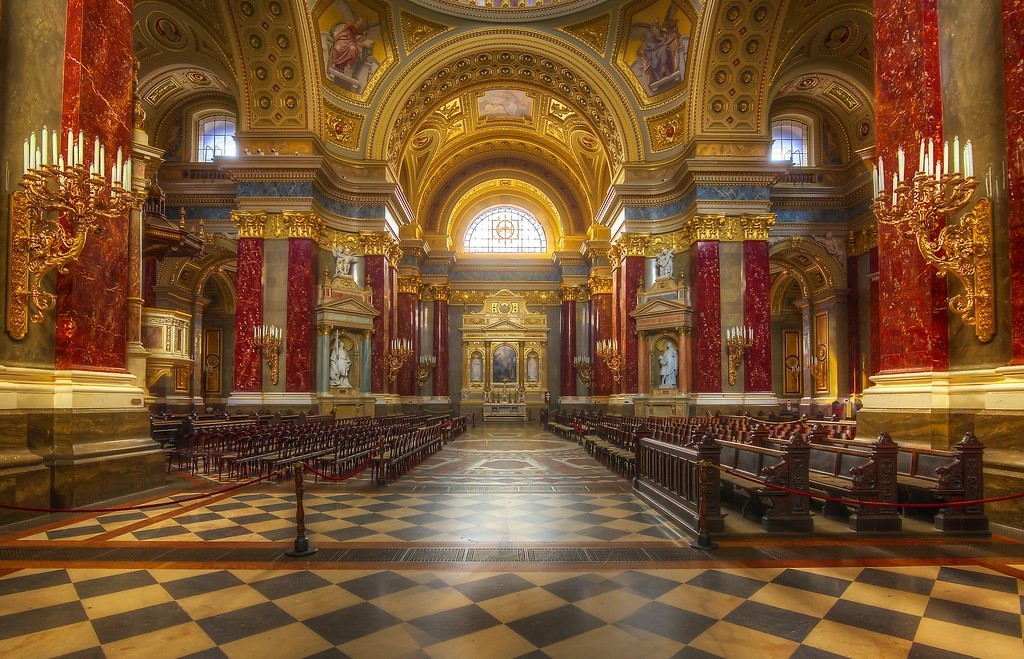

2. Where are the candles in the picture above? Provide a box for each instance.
[935,160,941,193]
[42,125,48,170]
[254,325,282,339]
[597,339,617,350]
[392,337,412,350]
[727,326,753,340]
[52,129,57,164]
[68,128,83,174]
[892,149,905,205]
[420,354,436,363]
[964,139,974,181]
[574,355,590,363]
[90,136,104,197]
[873,155,884,202]
[111,146,132,197]
[954,135,959,174]
[913,137,933,202]
[24,125,41,184]
[944,140,949,174]
[58,154,65,190]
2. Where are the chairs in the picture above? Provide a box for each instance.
[548,415,854,481]
[168,415,467,485]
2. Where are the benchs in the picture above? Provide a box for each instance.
[682,422,813,532]
[808,424,989,533]
[748,424,901,532]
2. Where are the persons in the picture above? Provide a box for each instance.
[336,246,352,275]
[330,342,353,388]
[656,249,675,276]
[659,342,679,385]
